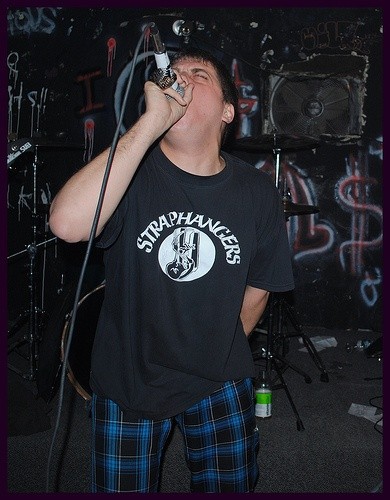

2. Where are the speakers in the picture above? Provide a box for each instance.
[264,62,365,142]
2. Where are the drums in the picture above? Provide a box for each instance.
[61,283,106,400]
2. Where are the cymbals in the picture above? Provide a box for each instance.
[225,133,321,154]
[282,199,320,216]
[32,137,85,147]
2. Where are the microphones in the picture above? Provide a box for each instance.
[150,23,185,101]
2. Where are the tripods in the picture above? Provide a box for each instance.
[247,138,329,432]
[3,105,58,382]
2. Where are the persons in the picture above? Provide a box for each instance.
[49,53,297,495]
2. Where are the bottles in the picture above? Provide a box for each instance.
[348,338,374,351]
[253,369,273,420]
